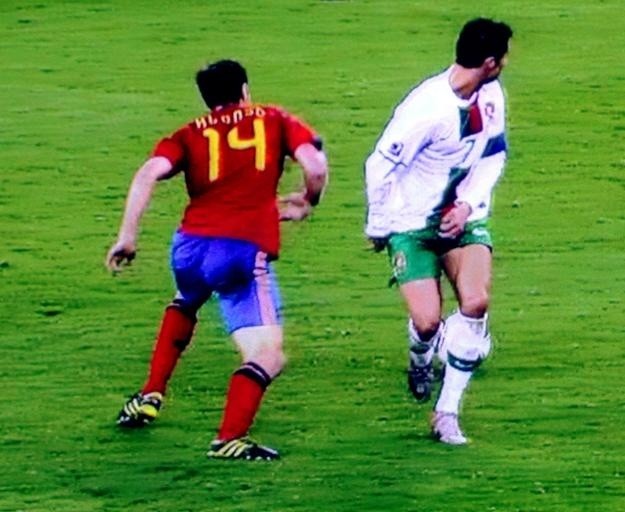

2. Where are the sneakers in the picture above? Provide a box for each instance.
[408,361,434,400]
[430,410,467,445]
[206,437,279,461]
[117,391,164,426]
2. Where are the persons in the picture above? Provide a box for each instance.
[365,19,513,445]
[106,59,327,460]
